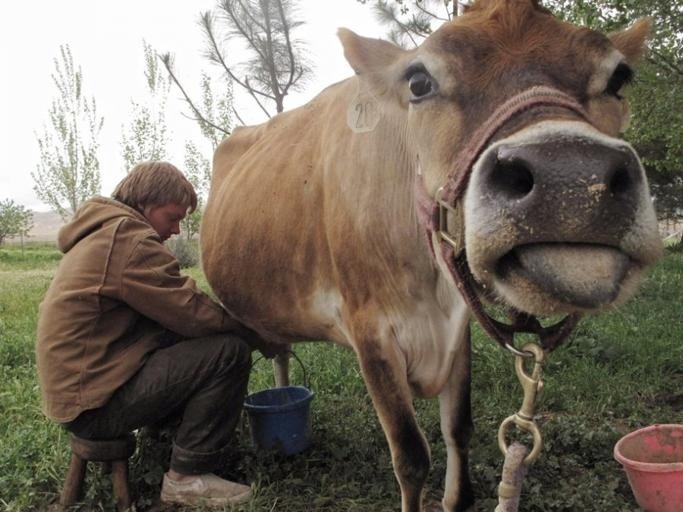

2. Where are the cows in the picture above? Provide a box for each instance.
[197,1,667,512]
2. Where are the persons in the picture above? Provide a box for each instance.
[36,158,276,510]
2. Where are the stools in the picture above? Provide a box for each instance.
[59,431,136,512]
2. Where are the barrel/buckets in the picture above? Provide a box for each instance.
[245,349,316,467]
[613,422,683,511]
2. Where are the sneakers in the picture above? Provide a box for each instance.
[162,473,253,506]
